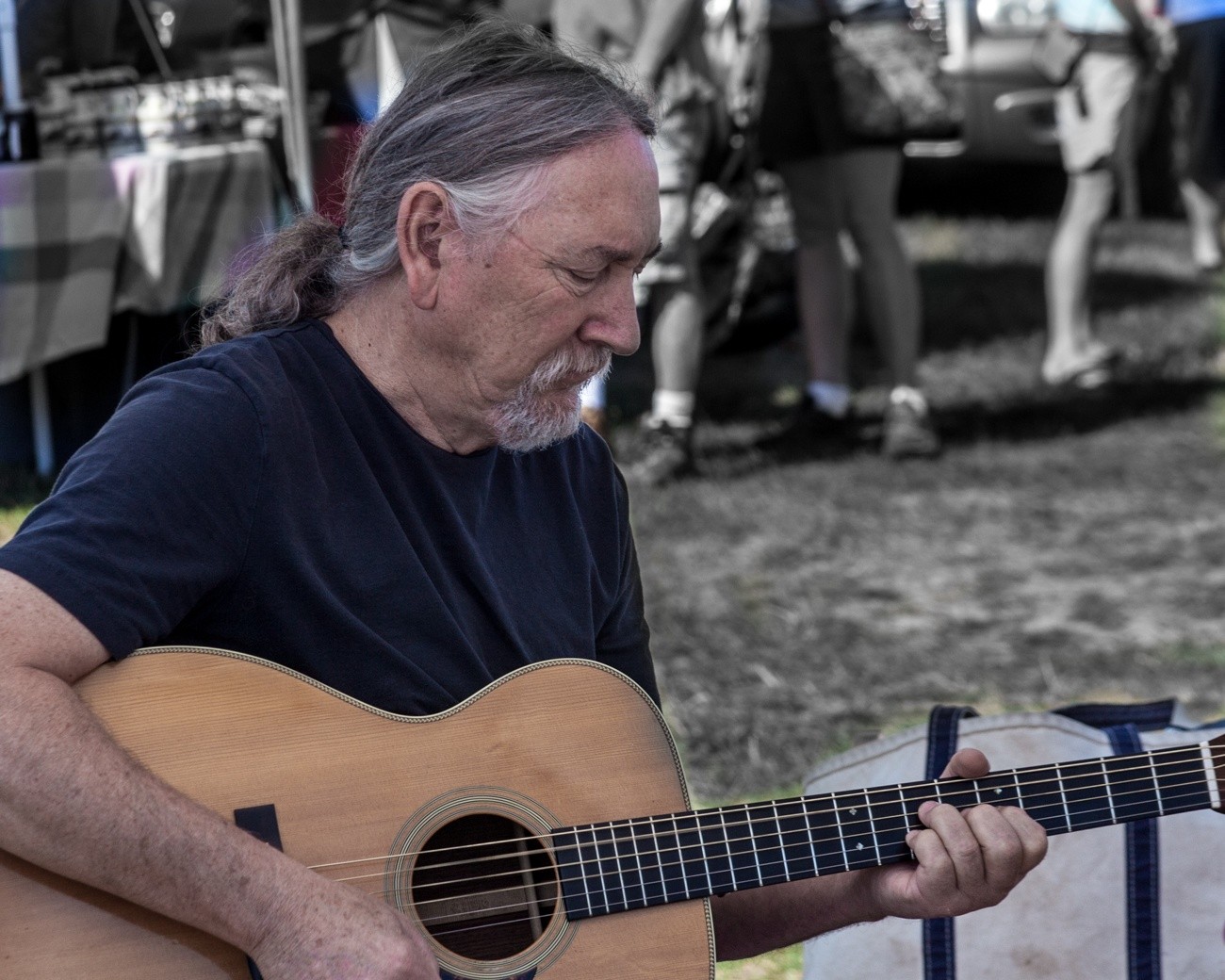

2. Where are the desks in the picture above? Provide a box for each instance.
[0,140,301,480]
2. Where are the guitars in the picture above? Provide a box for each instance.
[0,642,1224,978]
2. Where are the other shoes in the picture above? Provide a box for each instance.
[752,392,860,460]
[617,418,692,485]
[881,385,942,457]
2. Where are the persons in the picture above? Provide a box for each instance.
[0,21,1049,980]
[532,0,1225,487]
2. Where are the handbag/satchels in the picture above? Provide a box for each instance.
[1030,18,1083,87]
